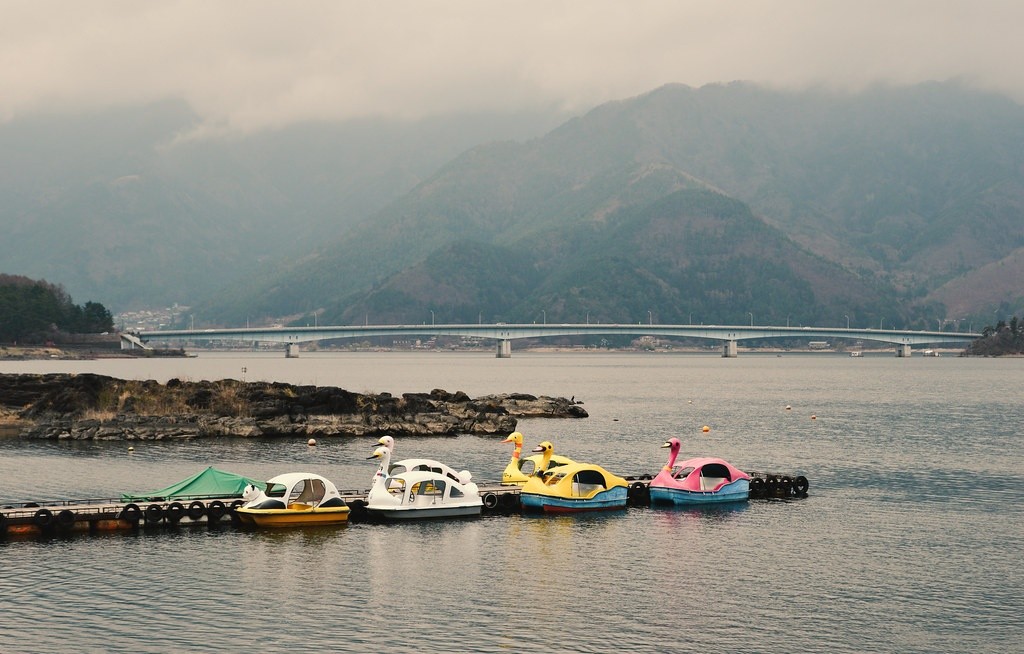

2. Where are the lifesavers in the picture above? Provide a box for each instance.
[631,482,647,502]
[482,493,499,509]
[779,476,793,495]
[751,477,766,494]
[56,510,76,531]
[765,474,779,494]
[188,501,206,520]
[146,504,163,522]
[639,473,652,480]
[24,502,39,508]
[351,499,367,516]
[229,500,245,517]
[35,508,54,528]
[208,501,226,520]
[793,475,810,495]
[500,493,517,509]
[122,503,142,525]
[167,502,186,522]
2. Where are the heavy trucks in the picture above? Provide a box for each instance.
[808,342,831,350]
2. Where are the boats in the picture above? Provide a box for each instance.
[649,438,752,505]
[850,351,864,356]
[350,436,485,524]
[233,472,349,524]
[499,432,579,490]
[521,441,629,513]
